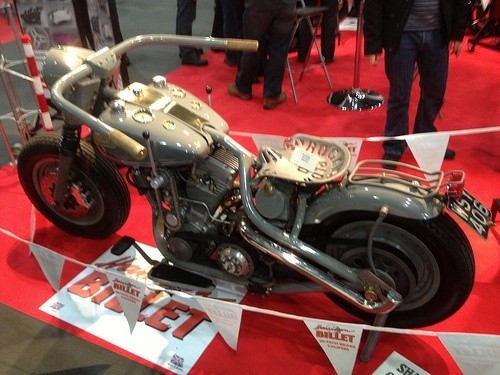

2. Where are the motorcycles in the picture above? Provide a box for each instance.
[0,33,492,361]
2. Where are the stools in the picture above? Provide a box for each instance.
[286,0,333,103]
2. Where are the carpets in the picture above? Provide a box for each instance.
[0,14,500,375]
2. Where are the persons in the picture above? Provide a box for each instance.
[362,0,471,170]
[296,0,339,62]
[176,0,268,83]
[228,0,296,109]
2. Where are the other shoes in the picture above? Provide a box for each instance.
[179,47,203,57]
[445,149,456,158]
[124,56,130,65]
[263,92,286,109]
[382,154,401,169]
[230,85,253,100]
[182,57,208,65]
